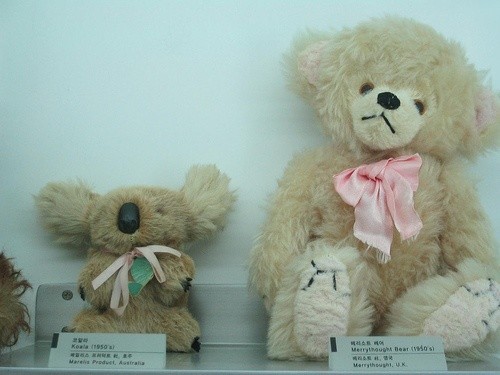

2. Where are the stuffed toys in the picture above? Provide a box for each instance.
[35,162,238,352]
[247,11,500,362]
